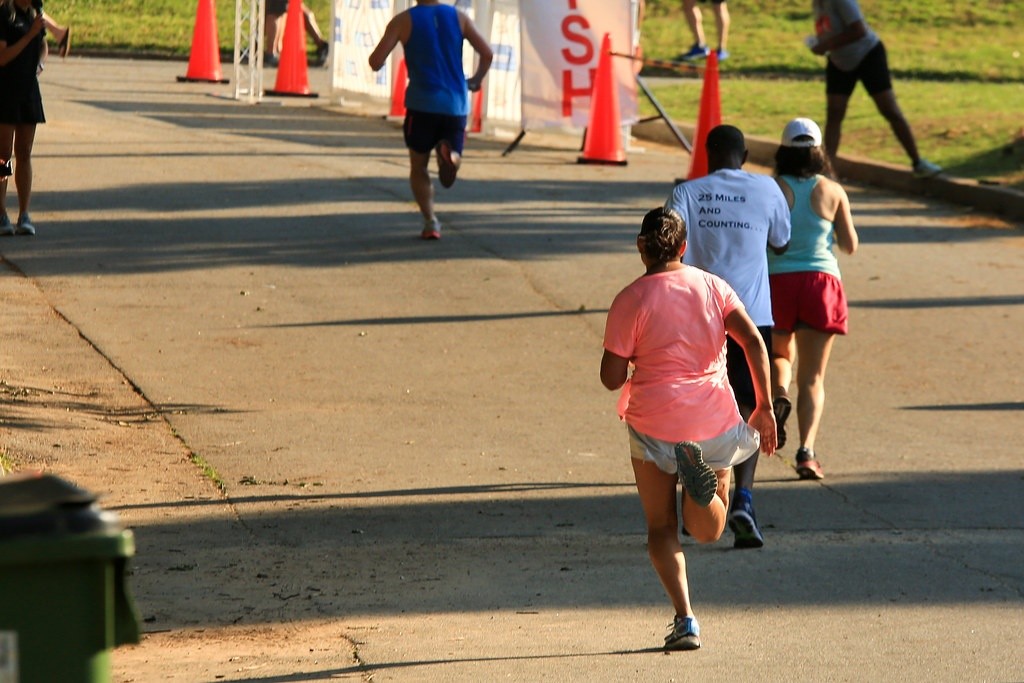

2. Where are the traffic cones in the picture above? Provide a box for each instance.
[391,59,409,116]
[675,50,722,184]
[576,33,628,167]
[470,84,483,133]
[176,0,229,85]
[264,0,319,98]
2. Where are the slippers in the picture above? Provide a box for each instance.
[59,26,69,59]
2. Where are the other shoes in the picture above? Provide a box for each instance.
[0,215,15,236]
[264,52,279,68]
[17,219,35,235]
[315,42,328,67]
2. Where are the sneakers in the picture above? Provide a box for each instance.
[677,43,710,62]
[436,139,457,189]
[663,615,701,649]
[728,488,764,548]
[422,220,441,239]
[715,50,730,61]
[795,447,825,479]
[675,440,718,508]
[913,159,941,179]
[773,396,792,450]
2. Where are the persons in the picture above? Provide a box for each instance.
[768,117,859,480]
[0,0,72,236]
[811,0,944,179]
[664,125,793,547]
[257,0,329,68]
[600,207,778,652]
[681,0,730,61]
[368,0,494,241]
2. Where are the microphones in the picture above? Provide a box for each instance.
[31,0,46,36]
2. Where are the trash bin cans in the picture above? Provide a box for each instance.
[0,475,140,683]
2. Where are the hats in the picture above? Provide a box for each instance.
[781,118,822,147]
